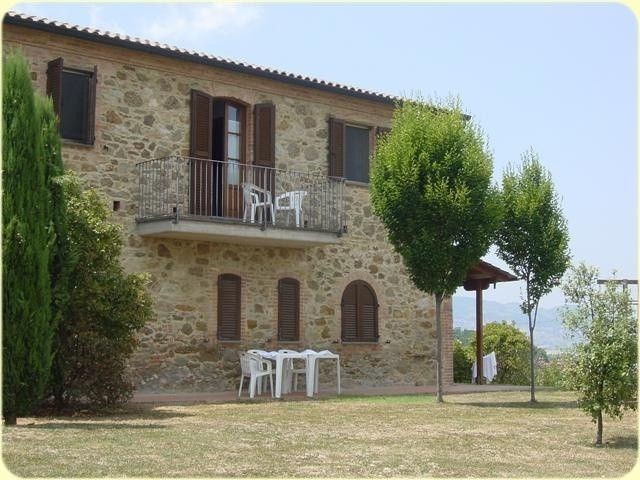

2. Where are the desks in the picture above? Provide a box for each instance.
[275,354,340,399]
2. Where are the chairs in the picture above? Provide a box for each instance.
[272,191,307,227]
[278,350,306,393]
[242,183,275,225]
[247,350,276,393]
[236,351,274,398]
